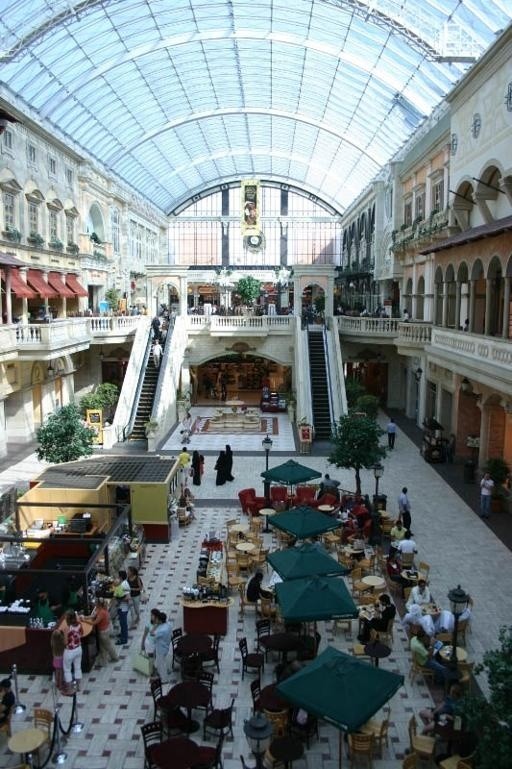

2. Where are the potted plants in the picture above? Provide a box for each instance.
[482,458,508,513]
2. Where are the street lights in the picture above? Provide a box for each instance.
[441,583,470,697]
[367,461,384,546]
[263,288,269,314]
[261,432,274,504]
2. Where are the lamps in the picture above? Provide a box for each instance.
[100,344,104,360]
[415,368,423,379]
[462,377,470,394]
[47,360,54,375]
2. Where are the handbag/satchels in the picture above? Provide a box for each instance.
[141,593,149,601]
[133,652,153,675]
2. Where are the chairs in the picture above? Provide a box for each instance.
[0,704,16,737]
[34,709,53,750]
[68,310,122,328]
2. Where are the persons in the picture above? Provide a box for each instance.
[359,303,386,318]
[150,306,177,370]
[50,304,147,319]
[187,301,325,325]
[26,444,234,695]
[402,309,409,323]
[338,304,344,314]
[317,416,493,667]
[245,570,272,604]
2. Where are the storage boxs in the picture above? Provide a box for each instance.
[70,513,90,533]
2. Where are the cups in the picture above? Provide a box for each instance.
[54,524,64,534]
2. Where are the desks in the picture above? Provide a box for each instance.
[465,442,479,456]
[8,728,43,769]
[421,439,441,462]
[260,400,287,412]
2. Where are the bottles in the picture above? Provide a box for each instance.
[29,617,45,629]
